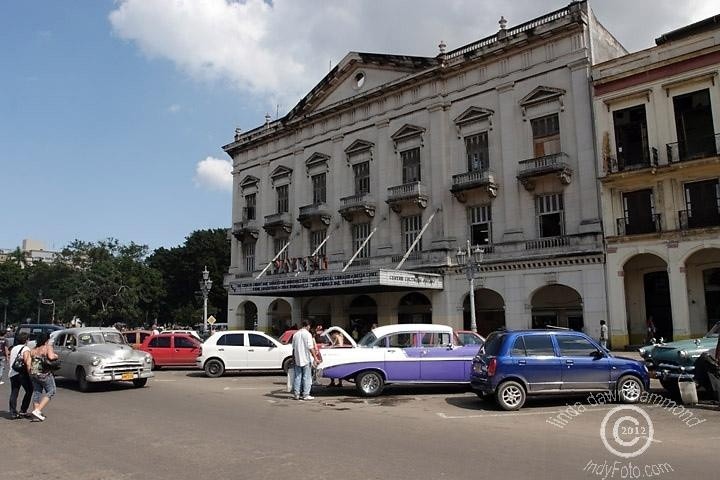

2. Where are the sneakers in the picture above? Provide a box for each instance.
[19,413,30,418]
[31,409,45,422]
[303,395,314,400]
[11,413,18,418]
[295,394,300,400]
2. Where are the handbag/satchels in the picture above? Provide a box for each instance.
[41,356,63,371]
[12,358,27,373]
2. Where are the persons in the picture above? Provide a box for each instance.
[112,321,228,343]
[285,318,380,400]
[598,319,608,350]
[0,323,58,422]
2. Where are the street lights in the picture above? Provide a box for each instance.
[456,240,488,336]
[197,265,214,333]
